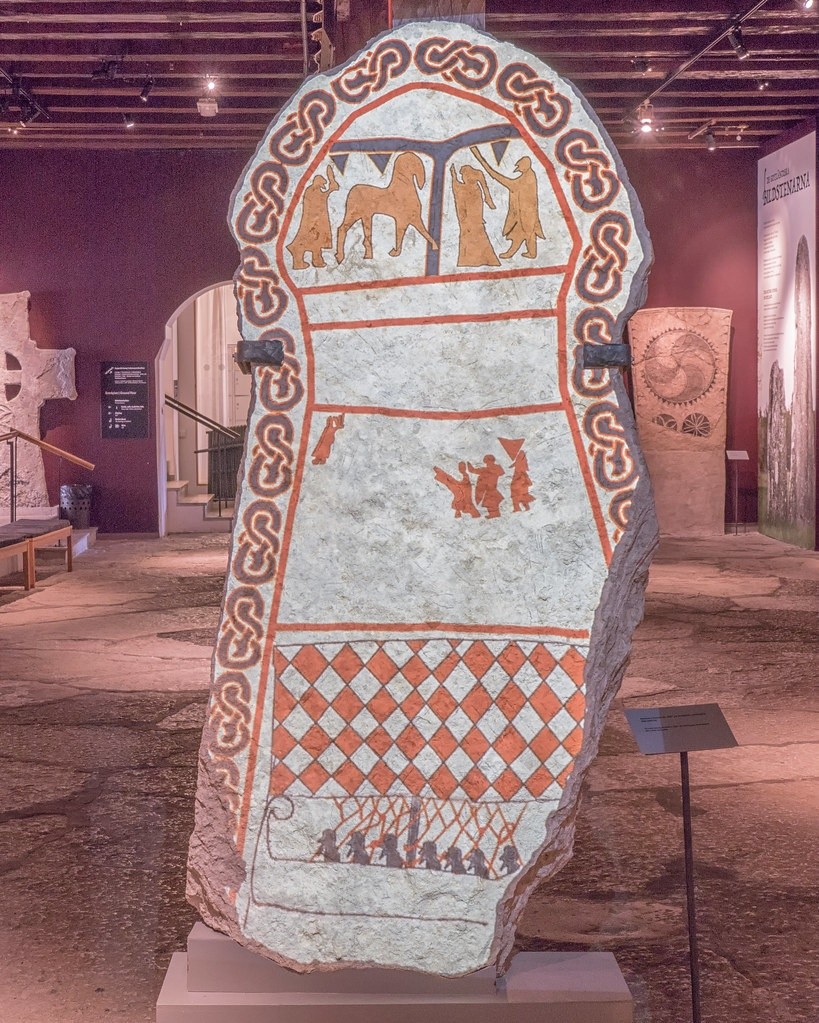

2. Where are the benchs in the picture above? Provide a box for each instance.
[0,518,74,590]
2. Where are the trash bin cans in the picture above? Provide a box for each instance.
[59,484,93,530]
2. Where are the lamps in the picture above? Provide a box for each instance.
[197,98,219,117]
[622,119,635,135]
[20,110,40,128]
[728,34,749,60]
[795,0,813,8]
[705,132,716,151]
[138,79,153,102]
[637,101,654,132]
[121,112,134,129]
[634,61,651,75]
